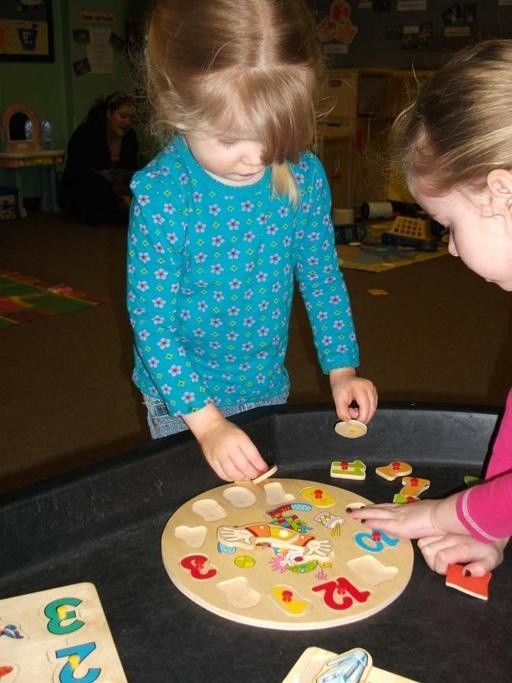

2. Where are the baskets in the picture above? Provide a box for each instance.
[386,215,436,242]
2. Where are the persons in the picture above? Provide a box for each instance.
[58,89,138,228]
[345,37,512,581]
[124,1,379,480]
